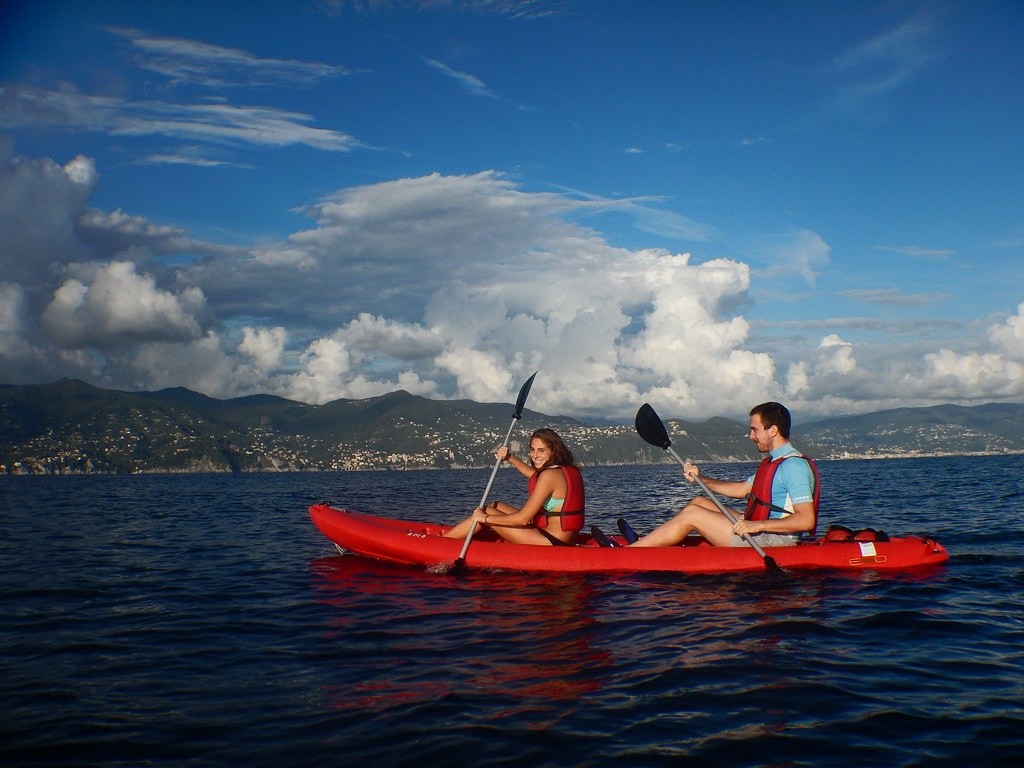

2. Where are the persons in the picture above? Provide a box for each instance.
[443,428,584,546]
[590,402,820,548]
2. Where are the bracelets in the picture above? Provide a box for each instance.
[484,514,491,523]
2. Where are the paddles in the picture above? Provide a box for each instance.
[634,402,785,575]
[447,370,540,570]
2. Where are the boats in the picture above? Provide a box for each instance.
[307,501,949,583]
